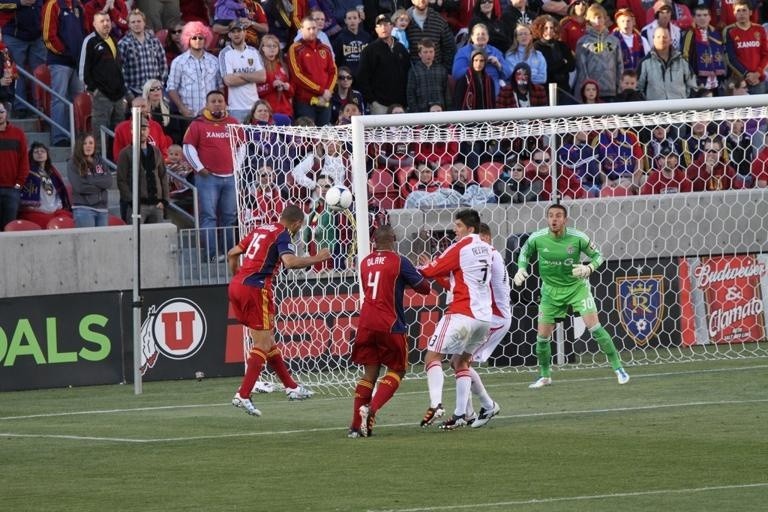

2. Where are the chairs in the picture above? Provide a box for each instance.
[0,43,767,232]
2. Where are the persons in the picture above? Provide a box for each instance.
[415,210,493,430]
[514,204,630,388]
[0,1,767,273]
[229,206,331,417]
[346,226,431,438]
[465,223,511,427]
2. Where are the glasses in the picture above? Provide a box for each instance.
[316,185,331,189]
[192,34,205,40]
[574,1,587,6]
[706,147,721,153]
[535,158,550,164]
[260,172,273,177]
[149,86,162,91]
[337,75,354,81]
[512,168,523,172]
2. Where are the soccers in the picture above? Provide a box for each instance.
[324,183,354,213]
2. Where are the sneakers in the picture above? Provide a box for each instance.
[420,403,446,429]
[231,391,263,418]
[438,413,468,432]
[347,428,360,438]
[466,411,478,424]
[285,385,314,401]
[528,376,552,389]
[613,366,631,384]
[15,110,27,118]
[359,404,375,438]
[54,139,71,147]
[471,401,500,428]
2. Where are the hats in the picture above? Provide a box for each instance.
[130,117,150,129]
[566,0,582,13]
[658,146,678,158]
[375,14,391,25]
[614,8,636,19]
[228,20,245,32]
[652,0,673,14]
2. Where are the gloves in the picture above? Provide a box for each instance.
[514,267,530,286]
[571,264,594,279]
